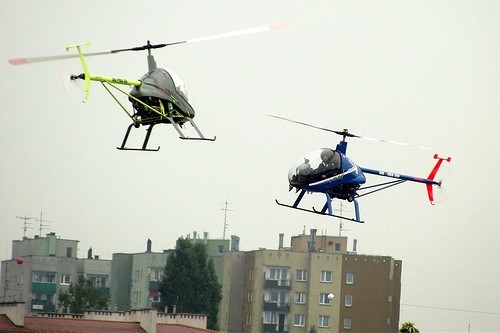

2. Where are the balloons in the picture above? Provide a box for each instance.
[15,256,25,265]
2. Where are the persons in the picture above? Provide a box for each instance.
[311,151,337,172]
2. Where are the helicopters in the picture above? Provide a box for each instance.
[6,22,288,152]
[262,113,452,223]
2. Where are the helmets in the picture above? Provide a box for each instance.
[320,150,333,159]
